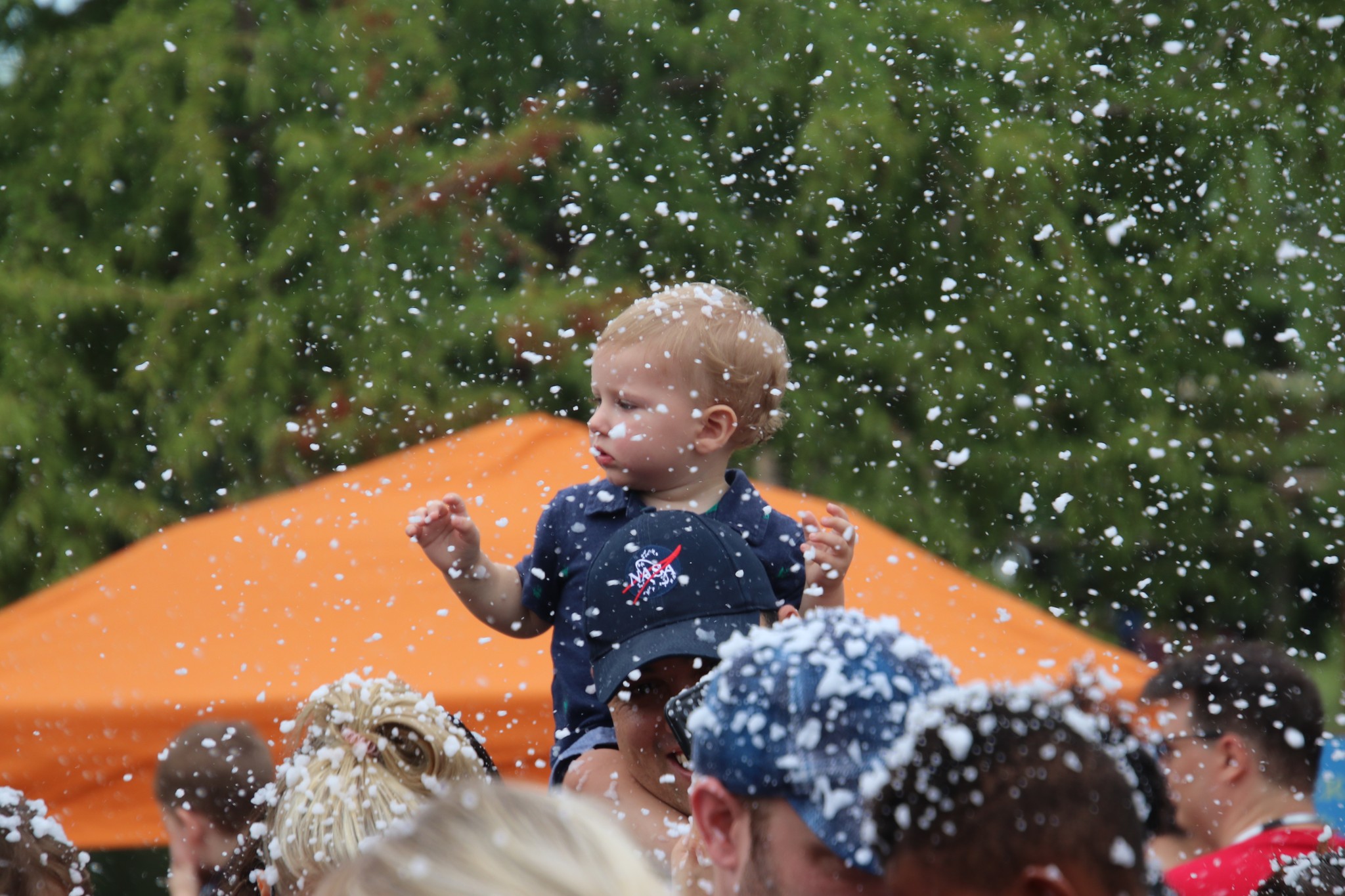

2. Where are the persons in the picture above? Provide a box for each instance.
[0,608,1345,896]
[409,280,858,882]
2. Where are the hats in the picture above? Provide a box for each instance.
[581,506,777,705]
[689,610,957,877]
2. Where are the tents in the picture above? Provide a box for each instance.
[0,413,1167,851]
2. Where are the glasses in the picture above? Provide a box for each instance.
[1147,730,1222,760]
